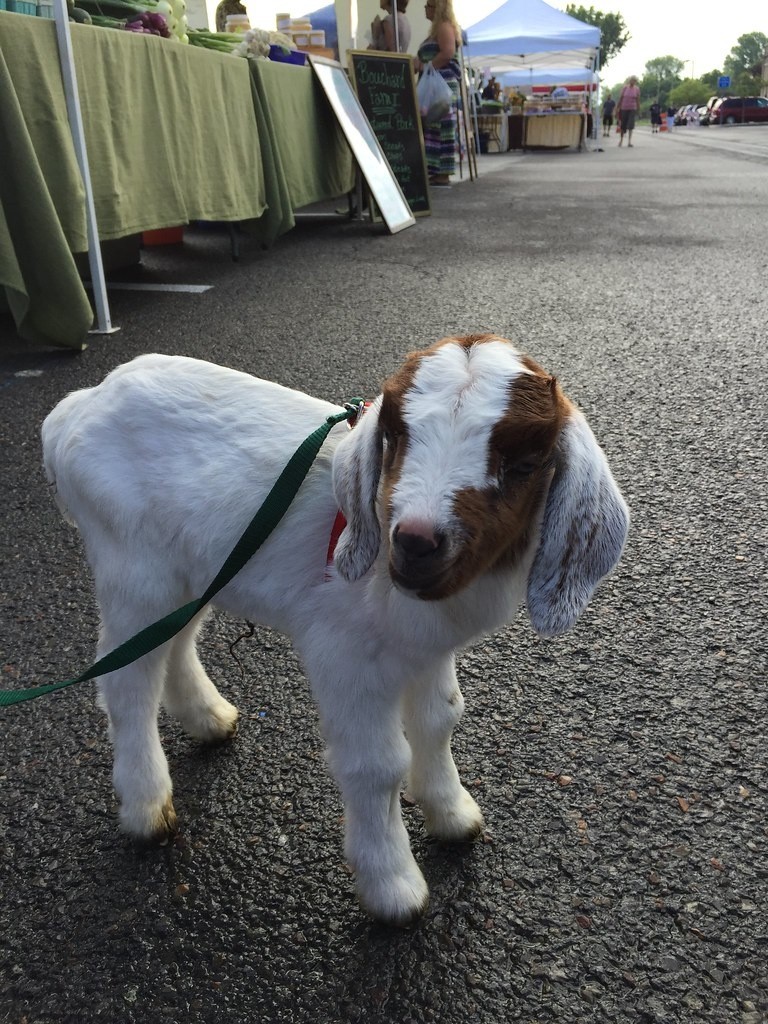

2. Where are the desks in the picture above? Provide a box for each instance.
[467,96,595,151]
[0,8,364,223]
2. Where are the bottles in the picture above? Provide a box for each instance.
[226,9,329,49]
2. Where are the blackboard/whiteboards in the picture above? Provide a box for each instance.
[307,53,416,234]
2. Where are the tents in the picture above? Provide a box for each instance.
[459,0,602,152]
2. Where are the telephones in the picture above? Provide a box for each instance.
[345,48,432,222]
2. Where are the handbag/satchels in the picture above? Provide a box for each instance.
[615,104,627,134]
[414,60,454,130]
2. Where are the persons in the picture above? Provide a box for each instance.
[665,102,681,133]
[617,77,641,147]
[600,92,615,138]
[649,99,662,134]
[409,0,462,189]
[372,1,413,53]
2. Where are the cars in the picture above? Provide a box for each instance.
[672,93,768,127]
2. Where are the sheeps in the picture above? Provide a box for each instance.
[42,335,630,925]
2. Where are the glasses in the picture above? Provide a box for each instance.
[424,2,436,9]
